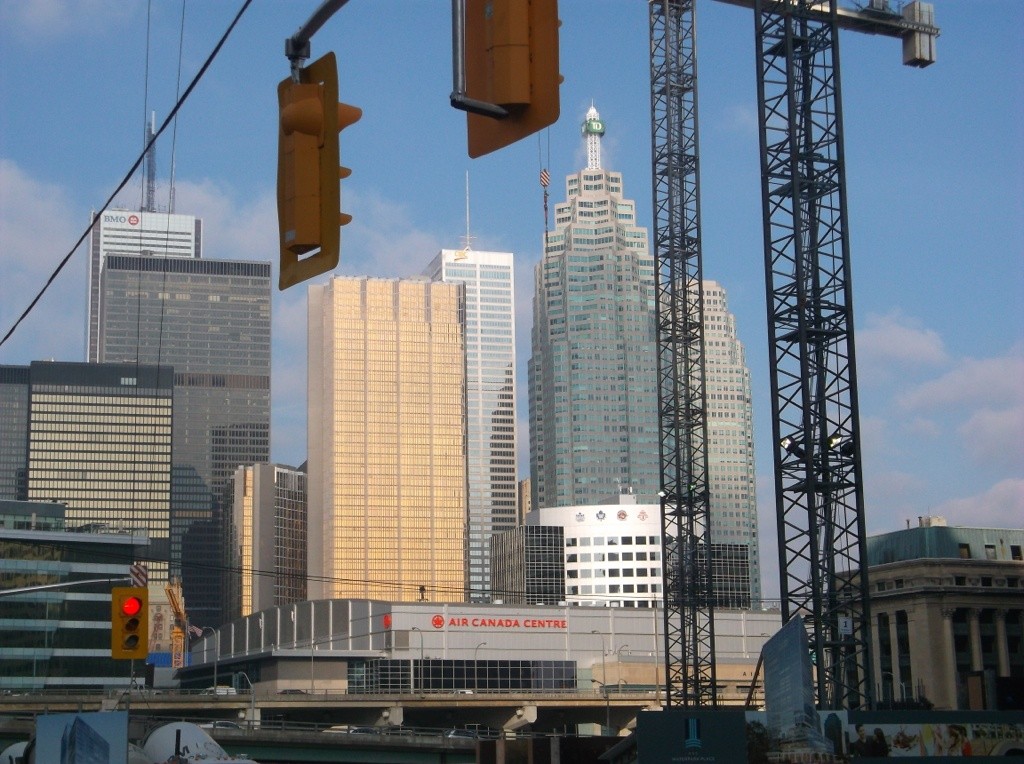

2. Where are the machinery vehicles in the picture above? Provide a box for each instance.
[0,721,257,764]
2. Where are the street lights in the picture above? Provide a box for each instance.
[883,672,906,704]
[202,626,217,695]
[412,627,425,694]
[591,679,610,737]
[592,630,606,686]
[474,643,488,694]
[617,645,629,694]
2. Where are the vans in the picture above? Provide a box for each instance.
[200,684,236,694]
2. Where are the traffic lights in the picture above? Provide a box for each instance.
[112,587,150,659]
[465,0,565,159]
[275,49,364,291]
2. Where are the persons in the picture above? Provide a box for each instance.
[853,723,874,757]
[872,727,889,757]
[974,726,988,753]
[749,745,769,764]
[934,725,944,752]
[945,725,972,756]
[892,730,911,750]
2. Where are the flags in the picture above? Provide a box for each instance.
[188,622,203,637]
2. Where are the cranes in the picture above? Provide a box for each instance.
[649,0,720,704]
[729,1,941,708]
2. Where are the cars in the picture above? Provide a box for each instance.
[277,689,308,694]
[116,684,163,695]
[449,689,473,695]
[197,721,240,730]
[320,726,386,736]
[437,728,485,739]
